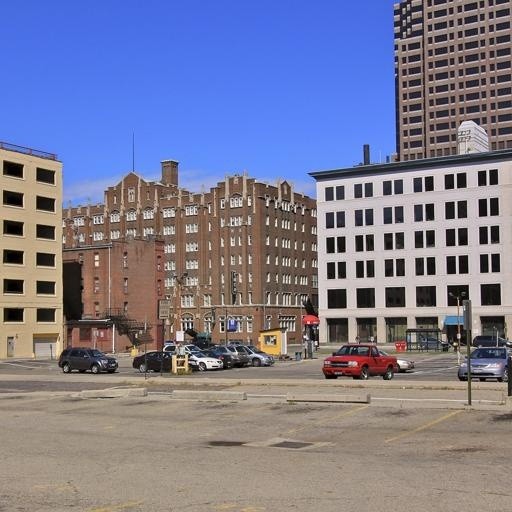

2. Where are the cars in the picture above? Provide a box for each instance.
[379,349,414,372]
[456,346,510,382]
[421,336,451,349]
[133,336,274,375]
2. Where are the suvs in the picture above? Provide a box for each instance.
[57,346,119,375]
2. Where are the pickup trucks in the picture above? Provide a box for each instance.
[321,343,398,380]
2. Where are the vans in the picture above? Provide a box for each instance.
[470,334,512,347]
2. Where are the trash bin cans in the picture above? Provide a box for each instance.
[295,352,302,361]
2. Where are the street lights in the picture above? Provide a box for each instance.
[492,325,500,347]
[448,292,462,368]
